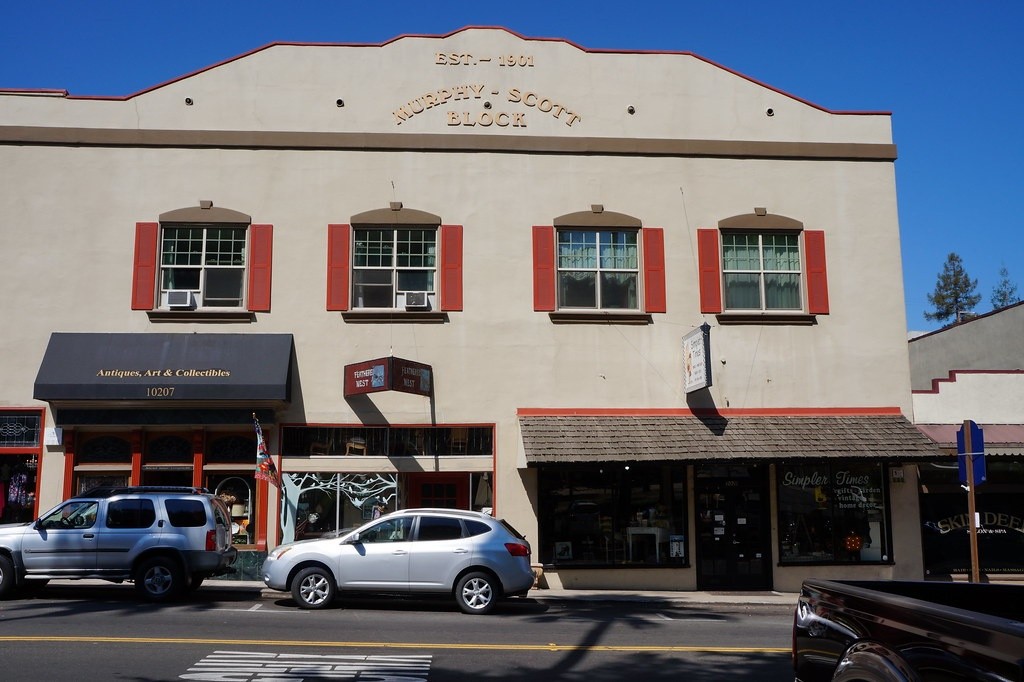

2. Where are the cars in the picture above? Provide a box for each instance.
[262,507,538,611]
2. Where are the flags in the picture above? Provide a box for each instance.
[254,418,278,488]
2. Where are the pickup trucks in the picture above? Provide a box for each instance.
[795,581,1024,682]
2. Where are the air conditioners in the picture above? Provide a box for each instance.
[405,292,427,307]
[166,290,194,307]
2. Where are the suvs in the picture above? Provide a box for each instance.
[0,484,238,607]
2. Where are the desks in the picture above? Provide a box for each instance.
[227,544,266,580]
[627,527,671,562]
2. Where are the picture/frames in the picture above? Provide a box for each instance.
[554,541,573,559]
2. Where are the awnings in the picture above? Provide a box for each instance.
[515,412,1024,469]
[34,332,292,399]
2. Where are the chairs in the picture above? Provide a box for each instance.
[346,433,372,455]
[598,515,626,563]
[449,429,468,455]
[310,429,335,455]
